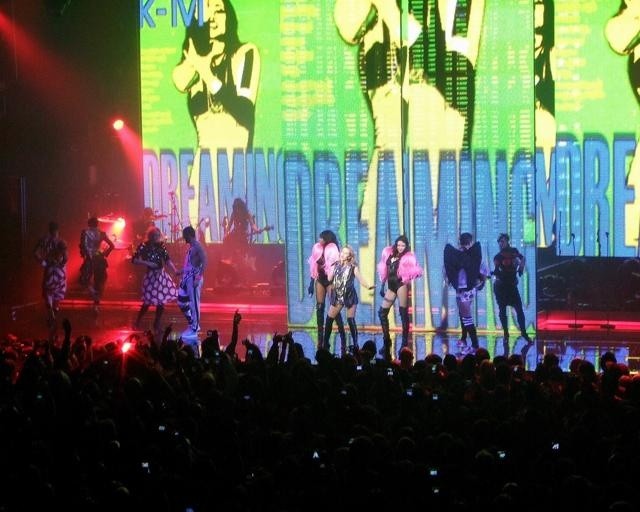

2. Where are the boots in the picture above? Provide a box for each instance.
[316,302,346,352]
[347,316,359,352]
[399,306,408,354]
[378,306,392,354]
[499,316,509,344]
[135,303,163,329]
[516,314,532,343]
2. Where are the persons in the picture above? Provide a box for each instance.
[33,218,115,325]
[225,198,259,269]
[143,208,155,241]
[444,233,485,355]
[309,230,376,351]
[490,233,533,344]
[173,226,206,336]
[376,236,422,356]
[131,227,177,332]
[1,309,639,512]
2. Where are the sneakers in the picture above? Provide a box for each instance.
[456,340,477,355]
[182,330,197,338]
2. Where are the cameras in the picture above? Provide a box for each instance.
[139,364,560,494]
